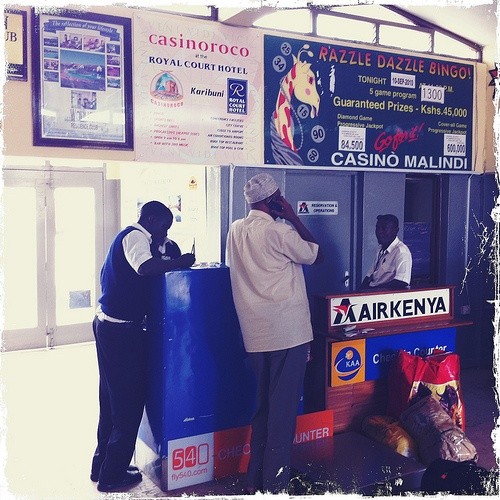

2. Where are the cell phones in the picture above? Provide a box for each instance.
[268,197,282,213]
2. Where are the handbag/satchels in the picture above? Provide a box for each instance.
[366,346,481,465]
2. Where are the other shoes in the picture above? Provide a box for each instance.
[90,464,142,492]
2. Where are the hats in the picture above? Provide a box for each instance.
[243,173,279,204]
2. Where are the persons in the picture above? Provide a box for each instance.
[44,34,120,88]
[137,202,143,219]
[359,214,412,289]
[78,96,96,109]
[167,199,181,222]
[90,200,196,491]
[157,230,182,261]
[225,173,323,496]
